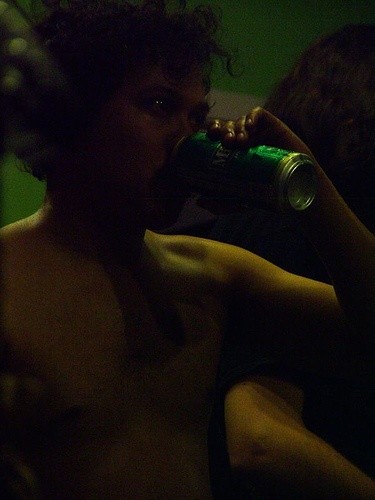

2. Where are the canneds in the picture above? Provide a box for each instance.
[171,128,319,212]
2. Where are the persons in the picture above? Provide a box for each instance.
[0,0,375,499]
[209,20,375,498]
